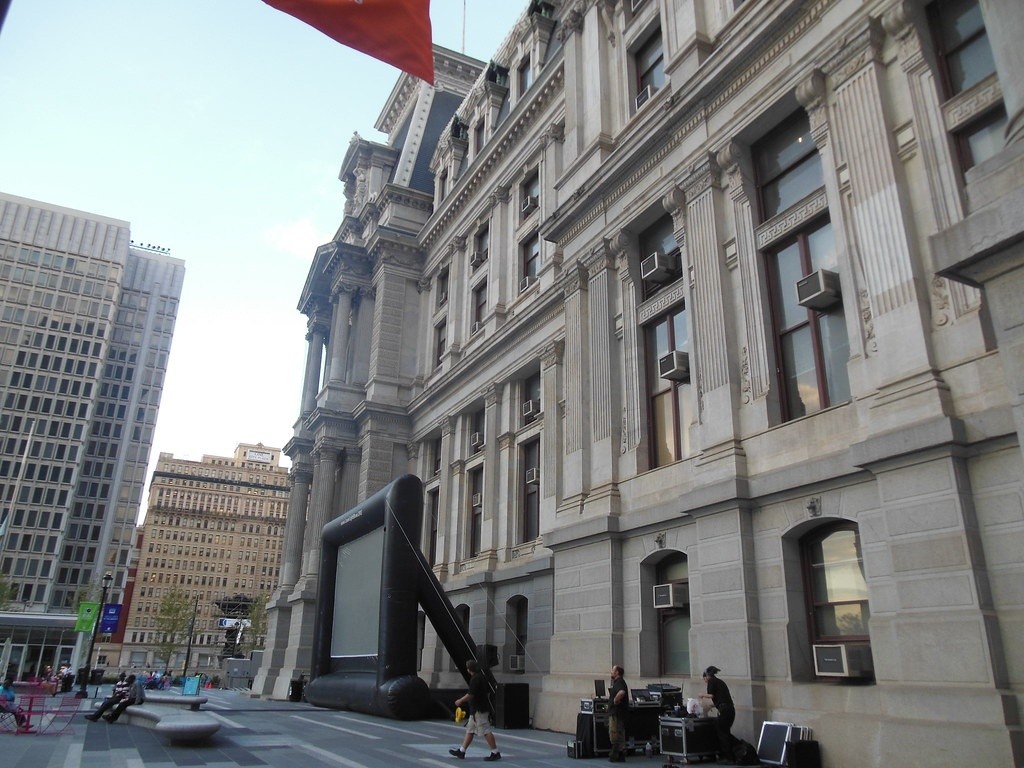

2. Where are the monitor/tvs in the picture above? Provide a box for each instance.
[595,680,605,698]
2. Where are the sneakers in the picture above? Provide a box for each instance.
[449,748,465,759]
[484,752,501,761]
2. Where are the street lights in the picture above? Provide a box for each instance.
[182,595,199,676]
[75,571,113,698]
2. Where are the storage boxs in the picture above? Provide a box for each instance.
[631,688,661,707]
[658,715,721,764]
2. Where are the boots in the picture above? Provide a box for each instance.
[616,751,625,762]
[609,743,619,762]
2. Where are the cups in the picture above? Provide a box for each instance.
[674,706,680,712]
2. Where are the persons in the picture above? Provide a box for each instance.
[85,673,130,722]
[102,675,145,724]
[608,665,628,762]
[698,666,736,765]
[0,677,33,729]
[146,670,173,690]
[62,664,72,676]
[449,660,501,761]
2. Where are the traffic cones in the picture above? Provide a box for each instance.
[204,682,212,688]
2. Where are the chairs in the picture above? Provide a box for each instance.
[0,694,81,737]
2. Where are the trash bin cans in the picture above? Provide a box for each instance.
[289,679,304,702]
[91,668,105,685]
[78,668,84,684]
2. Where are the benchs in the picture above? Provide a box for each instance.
[94,701,221,746]
[105,693,208,711]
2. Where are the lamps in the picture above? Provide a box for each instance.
[634,84,659,112]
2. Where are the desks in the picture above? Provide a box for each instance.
[17,695,46,733]
[576,706,687,758]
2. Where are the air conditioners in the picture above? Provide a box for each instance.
[794,268,842,309]
[470,251,486,267]
[520,276,537,293]
[658,350,690,381]
[522,196,538,213]
[471,432,484,447]
[439,292,447,303]
[472,493,482,506]
[526,468,540,485]
[640,251,676,283]
[523,400,540,417]
[813,644,874,677]
[471,321,485,336]
[652,584,689,608]
[508,655,525,670]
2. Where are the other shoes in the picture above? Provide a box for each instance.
[85,715,97,722]
[719,759,735,765]
[102,715,113,723]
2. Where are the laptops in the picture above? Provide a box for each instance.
[630,687,651,704]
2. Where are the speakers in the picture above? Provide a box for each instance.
[786,740,821,768]
[475,642,498,668]
[495,683,530,729]
